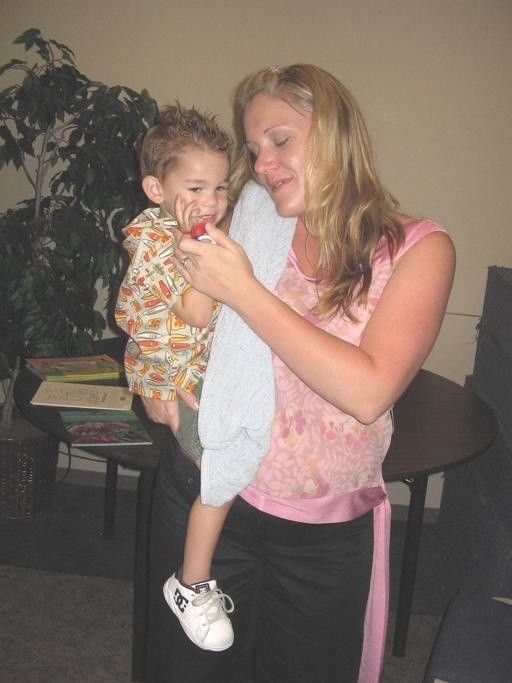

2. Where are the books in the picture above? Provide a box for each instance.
[59,408,153,447]
[26,354,122,382]
[30,379,134,410]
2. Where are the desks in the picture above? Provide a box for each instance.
[14,337,498,683]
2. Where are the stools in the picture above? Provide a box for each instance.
[425,589,512,683]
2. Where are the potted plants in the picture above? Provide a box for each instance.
[0,28,159,521]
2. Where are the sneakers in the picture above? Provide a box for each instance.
[162,572,235,652]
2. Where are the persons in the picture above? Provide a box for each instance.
[114,65,456,682]
